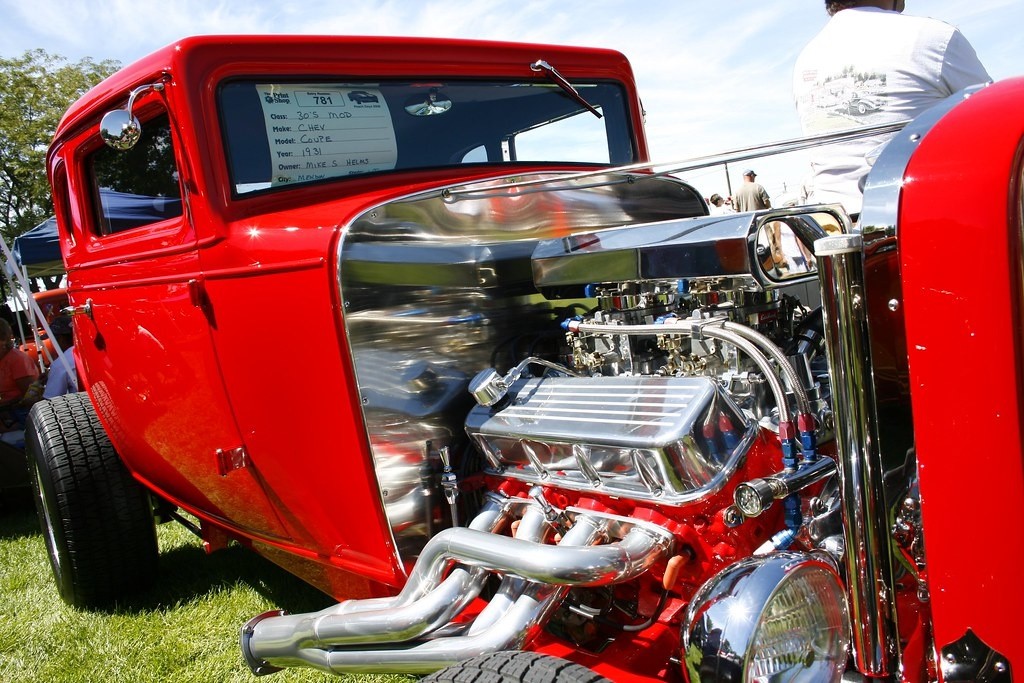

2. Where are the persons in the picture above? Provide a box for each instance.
[708,193,736,214]
[791,0,995,219]
[0,317,41,432]
[42,317,79,399]
[736,168,772,212]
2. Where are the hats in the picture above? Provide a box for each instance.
[744,169,757,176]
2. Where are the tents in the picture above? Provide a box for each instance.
[3,182,184,373]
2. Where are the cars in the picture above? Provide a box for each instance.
[0,27,1024,683]
